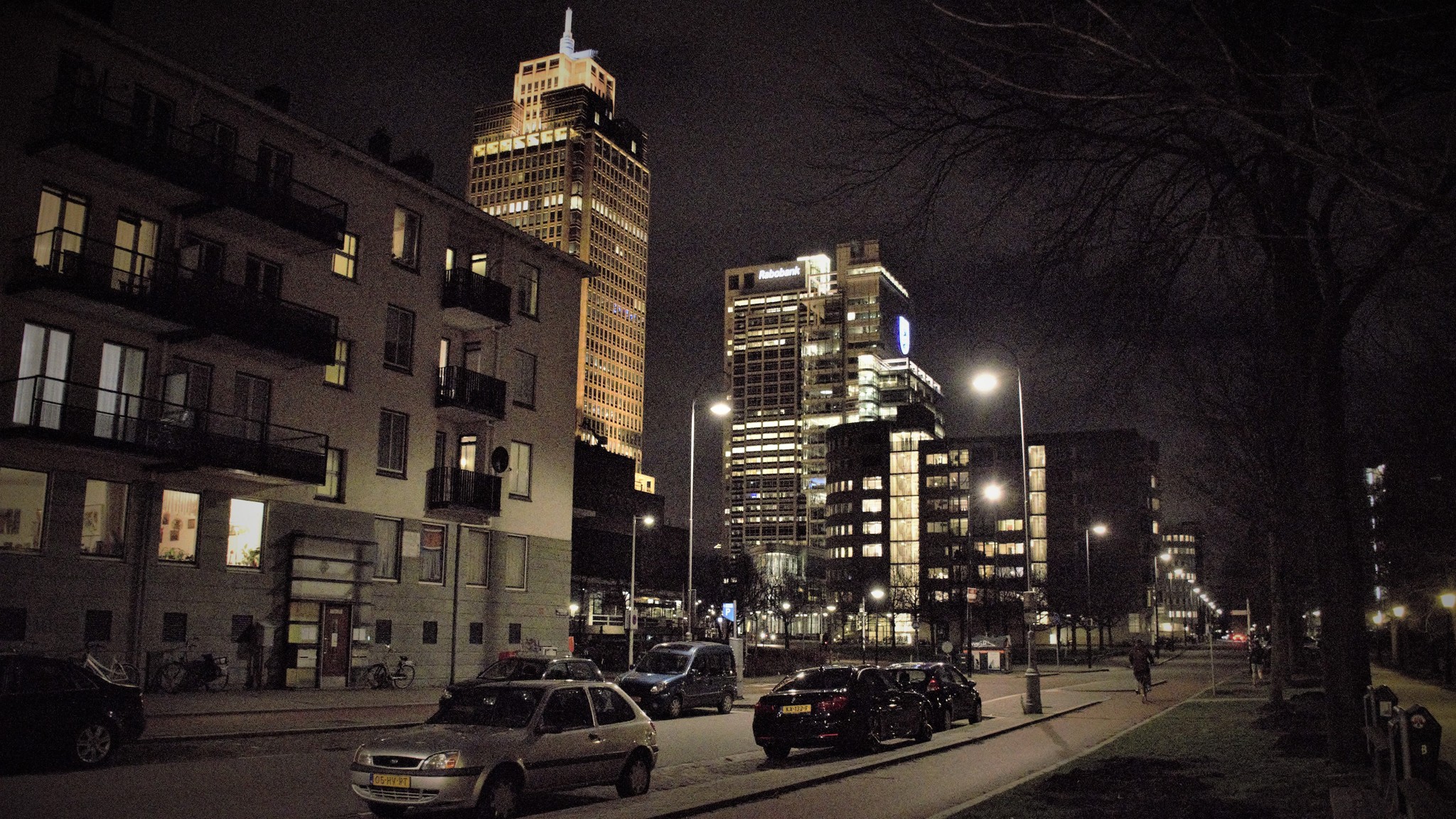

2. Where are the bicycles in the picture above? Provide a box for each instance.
[79,641,140,690]
[362,640,415,689]
[1130,662,1154,704]
[156,638,229,695]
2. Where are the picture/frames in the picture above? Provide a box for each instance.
[83,503,103,537]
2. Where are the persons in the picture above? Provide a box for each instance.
[1247,640,1266,687]
[1128,640,1153,695]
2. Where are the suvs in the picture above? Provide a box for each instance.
[620,641,740,720]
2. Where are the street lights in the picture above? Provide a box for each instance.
[1154,553,1223,660]
[688,393,732,639]
[629,514,654,671]
[1086,525,1107,668]
[862,588,884,664]
[966,338,1042,714]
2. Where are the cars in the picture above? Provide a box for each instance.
[0,653,146,770]
[438,654,608,719]
[885,662,983,733]
[350,678,659,819]
[751,664,934,760]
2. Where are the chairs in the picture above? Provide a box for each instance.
[899,672,910,682]
